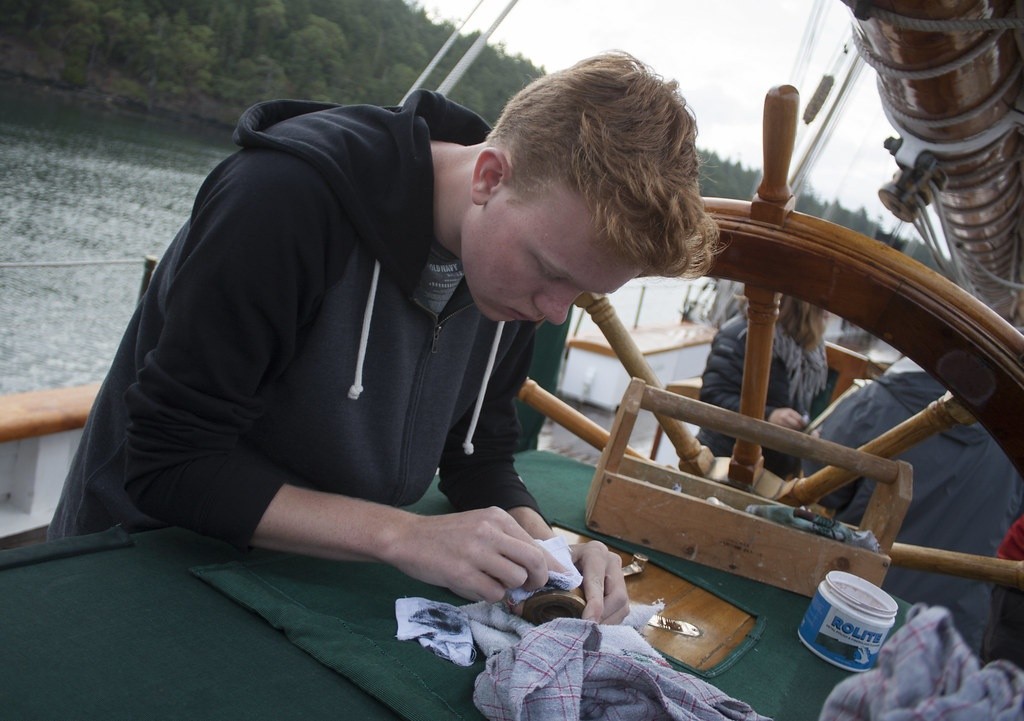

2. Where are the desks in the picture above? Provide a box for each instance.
[0,447,915,721]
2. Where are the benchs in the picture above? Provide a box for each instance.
[556,319,719,413]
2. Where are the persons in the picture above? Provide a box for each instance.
[799,357,1024,664]
[48,50,718,629]
[699,296,830,479]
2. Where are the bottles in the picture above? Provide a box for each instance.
[797,570,899,673]
